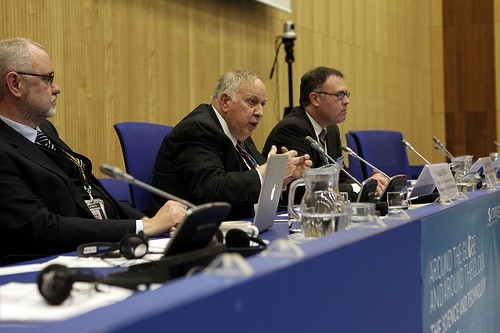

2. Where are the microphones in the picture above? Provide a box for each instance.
[340,143,393,178]
[402,139,429,164]
[432,136,453,157]
[98,163,225,242]
[433,145,453,159]
[305,135,361,186]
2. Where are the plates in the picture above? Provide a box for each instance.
[249,239,270,249]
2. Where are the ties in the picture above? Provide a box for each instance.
[235,141,257,170]
[317,129,327,153]
[35,131,84,171]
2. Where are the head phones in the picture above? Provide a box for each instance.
[78,231,149,259]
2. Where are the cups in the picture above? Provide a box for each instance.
[219,221,258,245]
[288,205,302,234]
[387,192,409,214]
[340,191,375,230]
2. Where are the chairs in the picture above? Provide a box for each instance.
[347,130,412,184]
[113,121,174,214]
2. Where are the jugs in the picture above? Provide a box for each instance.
[288,163,347,241]
[448,154,477,194]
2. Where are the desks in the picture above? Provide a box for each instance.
[0,182,500,333]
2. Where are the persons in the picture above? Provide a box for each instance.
[261,66,390,206]
[1,38,188,265]
[152,68,313,221]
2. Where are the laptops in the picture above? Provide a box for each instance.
[254,154,289,233]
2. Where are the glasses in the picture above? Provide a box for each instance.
[6,72,54,84]
[314,91,350,100]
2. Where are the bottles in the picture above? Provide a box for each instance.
[490,152,500,189]
